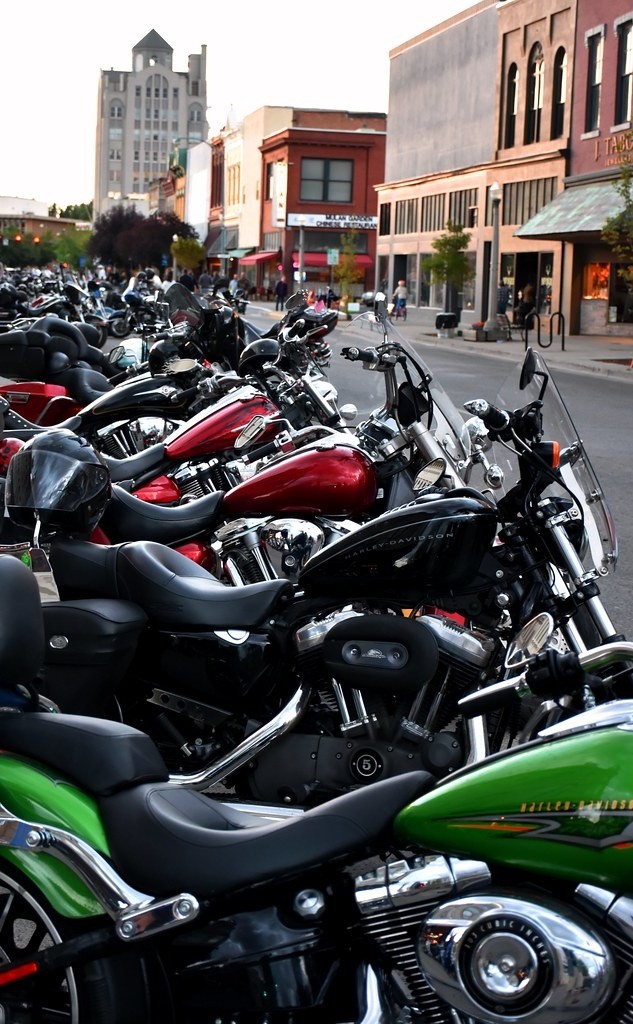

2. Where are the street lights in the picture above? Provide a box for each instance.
[483,180,503,333]
[297,214,306,290]
[172,233,180,281]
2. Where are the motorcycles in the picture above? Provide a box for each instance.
[0,249,359,507]
[1,339,632,814]
[0,552,632,1024]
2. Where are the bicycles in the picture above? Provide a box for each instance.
[393,294,407,322]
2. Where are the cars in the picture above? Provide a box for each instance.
[361,279,388,308]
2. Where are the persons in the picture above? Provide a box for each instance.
[522,282,536,322]
[3,259,338,310]
[495,281,510,318]
[393,278,408,313]
